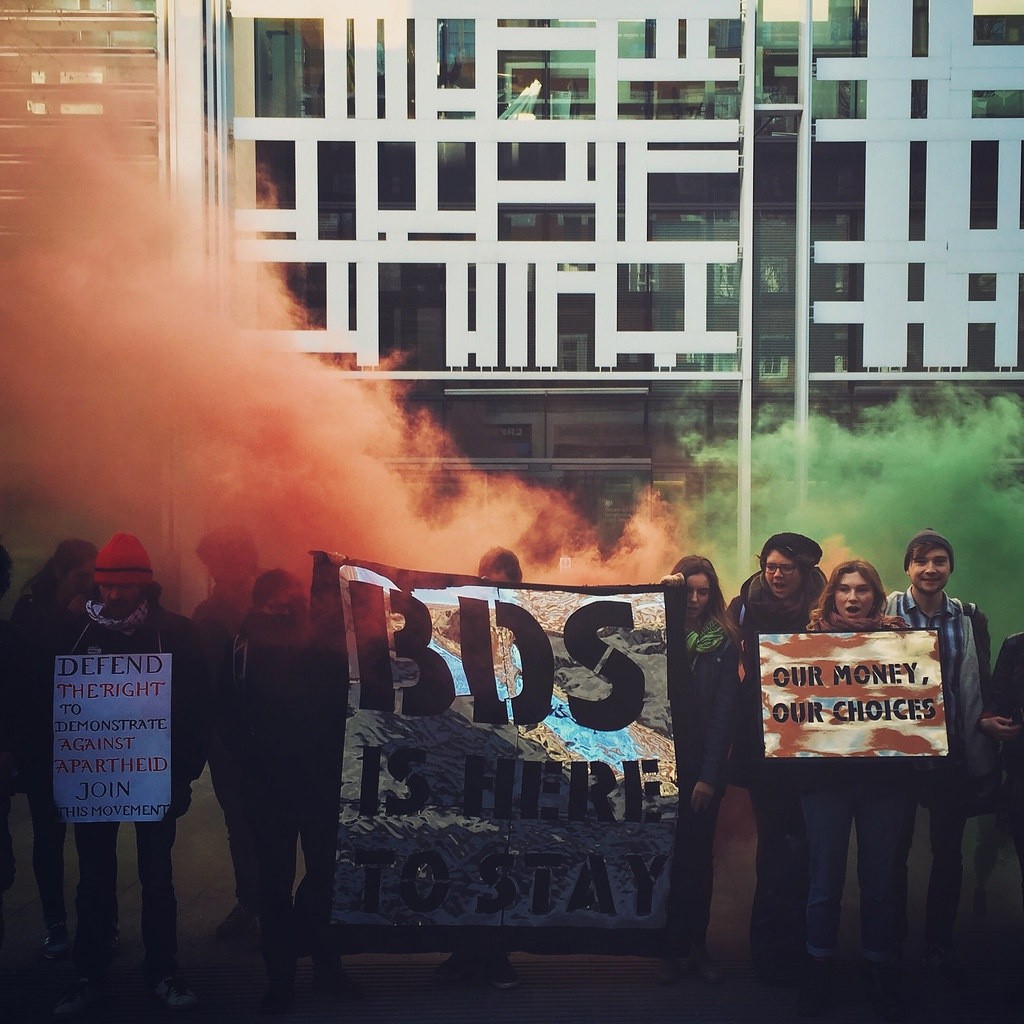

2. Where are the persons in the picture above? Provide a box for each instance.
[0,524,373,1010]
[660,531,1023,989]
[440,547,526,989]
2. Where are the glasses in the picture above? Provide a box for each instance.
[761,562,798,575]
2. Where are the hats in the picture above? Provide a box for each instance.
[94,532,154,588]
[904,528,955,573]
[760,533,822,572]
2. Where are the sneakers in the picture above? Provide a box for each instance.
[147,971,197,1010]
[52,976,95,1021]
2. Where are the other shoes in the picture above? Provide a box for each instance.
[654,955,680,982]
[218,904,252,940]
[753,959,792,986]
[874,979,904,1023]
[261,976,291,1014]
[312,969,366,998]
[43,921,69,958]
[796,967,831,1016]
[691,944,724,985]
[430,951,473,986]
[477,945,519,989]
[111,918,121,955]
